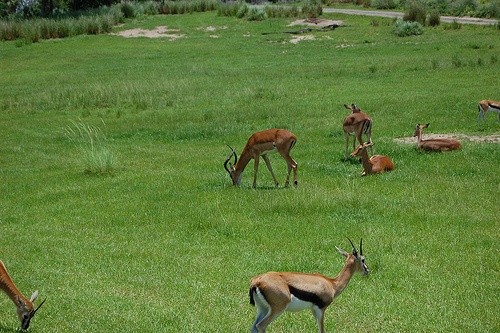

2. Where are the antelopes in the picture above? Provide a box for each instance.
[413,123,463,152]
[0,260,47,331]
[342,103,375,162]
[223,128,299,191]
[349,140,395,177]
[476,99,500,128]
[248,235,371,333]
